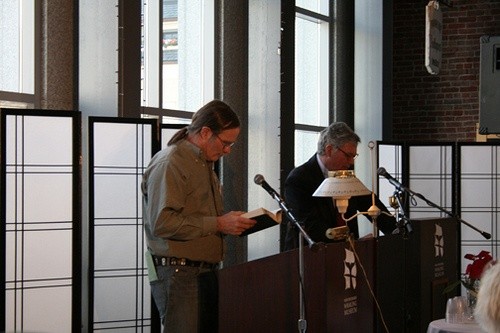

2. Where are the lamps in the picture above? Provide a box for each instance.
[312,141,400,333]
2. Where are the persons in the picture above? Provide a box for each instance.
[141,100,256,333]
[280,122,398,253]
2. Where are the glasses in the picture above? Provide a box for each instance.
[215,134,235,148]
[336,146,359,160]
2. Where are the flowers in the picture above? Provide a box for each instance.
[444,250,493,306]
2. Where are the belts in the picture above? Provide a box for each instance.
[152,256,219,270]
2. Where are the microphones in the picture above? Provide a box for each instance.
[376,167,407,192]
[254,174,287,208]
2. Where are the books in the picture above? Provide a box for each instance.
[240,208,283,237]
